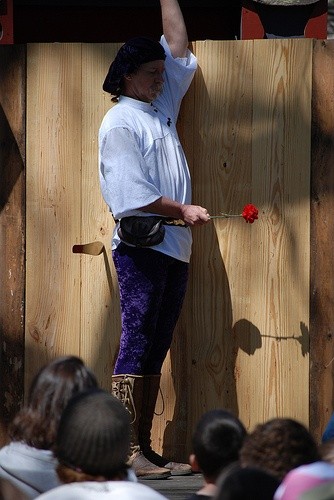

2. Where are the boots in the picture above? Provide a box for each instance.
[140,376,192,475]
[111,374,170,480]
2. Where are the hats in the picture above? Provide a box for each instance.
[104,37,166,93]
[273,462,334,500]
[321,414,334,441]
[56,390,130,476]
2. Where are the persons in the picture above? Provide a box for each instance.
[96,0,211,479]
[0,356,334,500]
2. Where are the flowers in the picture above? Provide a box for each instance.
[210,204,260,224]
